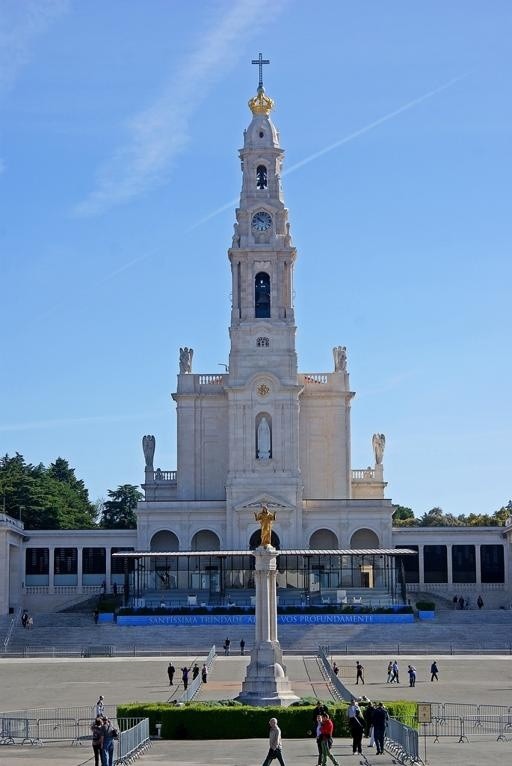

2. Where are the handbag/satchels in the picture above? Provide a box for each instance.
[112,728,120,740]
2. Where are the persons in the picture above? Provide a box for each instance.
[263,718,285,766]
[452,593,484,610]
[90,718,104,766]
[201,663,208,683]
[100,716,115,766]
[333,346,346,370]
[180,666,191,690]
[312,698,390,766]
[110,582,118,597]
[373,433,385,463]
[96,696,104,718]
[333,661,338,676]
[256,416,272,454]
[21,611,34,631]
[224,636,230,655]
[430,660,439,681]
[354,660,364,684]
[168,662,175,685]
[191,663,199,679]
[390,659,399,683]
[386,661,396,682]
[99,583,104,598]
[92,607,99,624]
[407,664,416,687]
[143,434,153,465]
[253,504,277,546]
[179,347,194,375]
[240,637,246,655]
